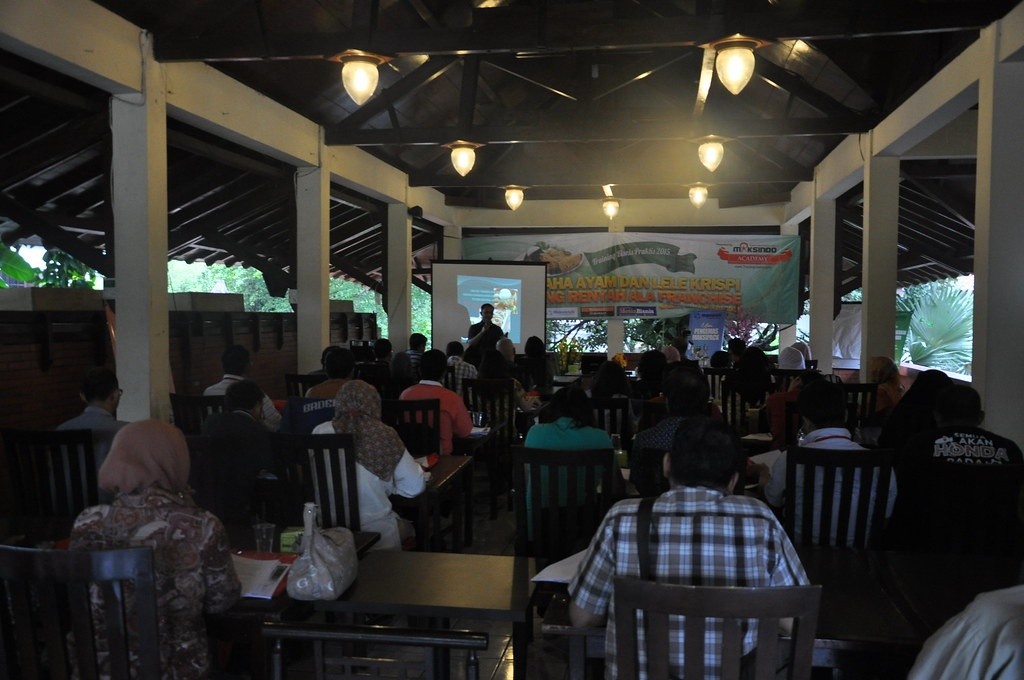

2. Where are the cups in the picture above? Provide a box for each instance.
[473,411,484,427]
[252,524,276,552]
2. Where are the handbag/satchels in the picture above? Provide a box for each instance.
[288,503,358,601]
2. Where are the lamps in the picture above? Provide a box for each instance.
[440,136,485,175]
[698,35,776,98]
[688,130,732,174]
[498,183,533,212]
[602,197,620,220]
[325,46,394,107]
[681,181,714,208]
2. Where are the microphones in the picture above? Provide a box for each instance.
[485,316,491,322]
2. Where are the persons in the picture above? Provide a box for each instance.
[47,370,130,511]
[907,584,1024,680]
[68,419,243,680]
[567,417,809,680]
[204,333,555,627]
[524,342,1024,553]
[468,303,508,349]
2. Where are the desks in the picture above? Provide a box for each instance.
[277,444,474,549]
[230,552,540,680]
[449,404,514,515]
[876,547,1023,634]
[551,375,579,387]
[541,538,931,680]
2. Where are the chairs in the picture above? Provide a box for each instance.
[0,358,1023,680]
[518,392,551,428]
[539,385,562,399]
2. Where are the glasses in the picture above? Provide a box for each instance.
[117,389,123,396]
